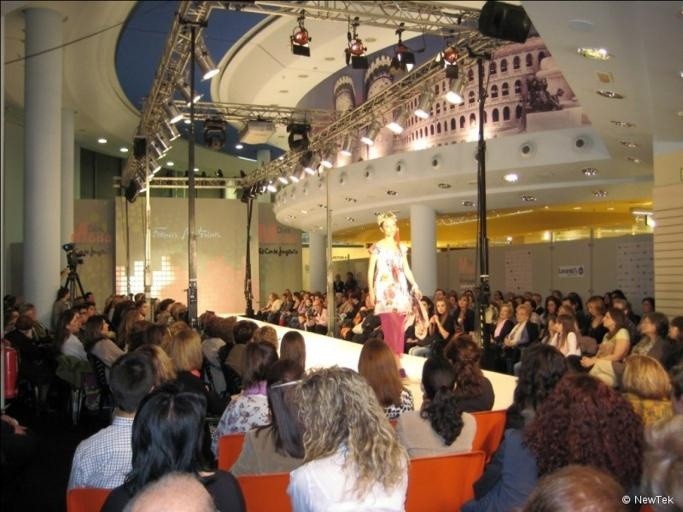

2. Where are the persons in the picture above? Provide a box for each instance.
[368,213,422,361]
[1,273,683,512]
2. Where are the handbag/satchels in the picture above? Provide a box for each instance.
[73,371,104,425]
[57,353,95,390]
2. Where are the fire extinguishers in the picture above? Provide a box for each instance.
[4,339,18,399]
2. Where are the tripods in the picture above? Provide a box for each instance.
[65,272,88,306]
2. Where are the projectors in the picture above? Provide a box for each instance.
[240,120,273,144]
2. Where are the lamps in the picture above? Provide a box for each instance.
[152,129,172,154]
[413,87,437,120]
[161,116,183,140]
[360,120,381,146]
[177,79,204,108]
[344,22,370,69]
[181,169,226,186]
[388,31,415,71]
[388,103,410,135]
[340,133,357,156]
[286,131,308,152]
[193,42,220,80]
[125,138,166,205]
[291,14,311,55]
[240,156,320,200]
[161,98,183,124]
[59,408,517,512]
[319,146,338,168]
[444,66,470,105]
